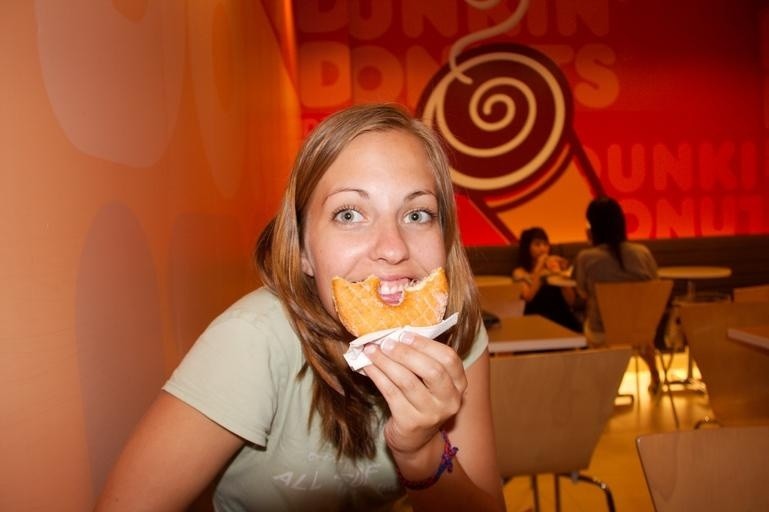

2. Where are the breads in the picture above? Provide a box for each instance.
[330,266,450,339]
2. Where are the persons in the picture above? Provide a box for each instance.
[512,225,585,337]
[576,193,664,401]
[93,103,511,510]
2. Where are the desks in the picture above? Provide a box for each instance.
[471,310,590,359]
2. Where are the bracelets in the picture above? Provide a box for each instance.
[398,427,461,490]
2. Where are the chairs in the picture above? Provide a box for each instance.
[672,300,765,429]
[481,340,635,511]
[632,427,766,509]
[587,281,683,429]
